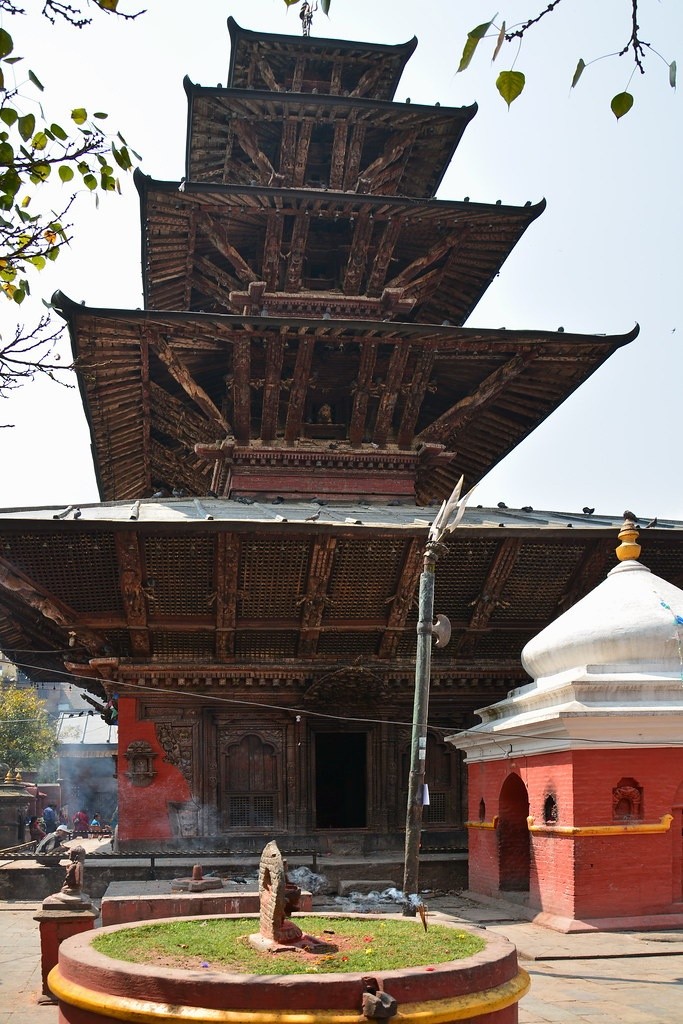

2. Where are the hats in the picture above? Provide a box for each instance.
[56,825,72,833]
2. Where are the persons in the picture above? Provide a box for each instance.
[41,803,60,852]
[72,808,90,840]
[28,816,46,844]
[91,815,100,827]
